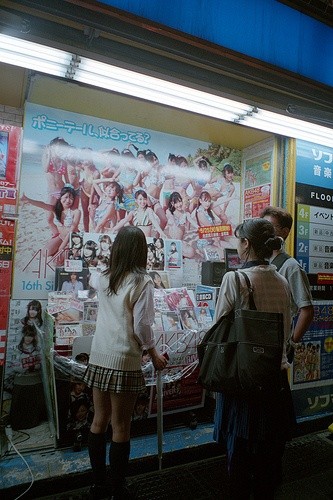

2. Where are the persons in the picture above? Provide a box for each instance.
[211,217,299,499]
[83,226,170,500]
[19,135,236,432]
[256,206,313,364]
[293,340,321,385]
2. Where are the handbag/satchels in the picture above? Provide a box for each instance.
[196,269,289,398]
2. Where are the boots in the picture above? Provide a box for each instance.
[88,431,112,500]
[109,438,137,500]
